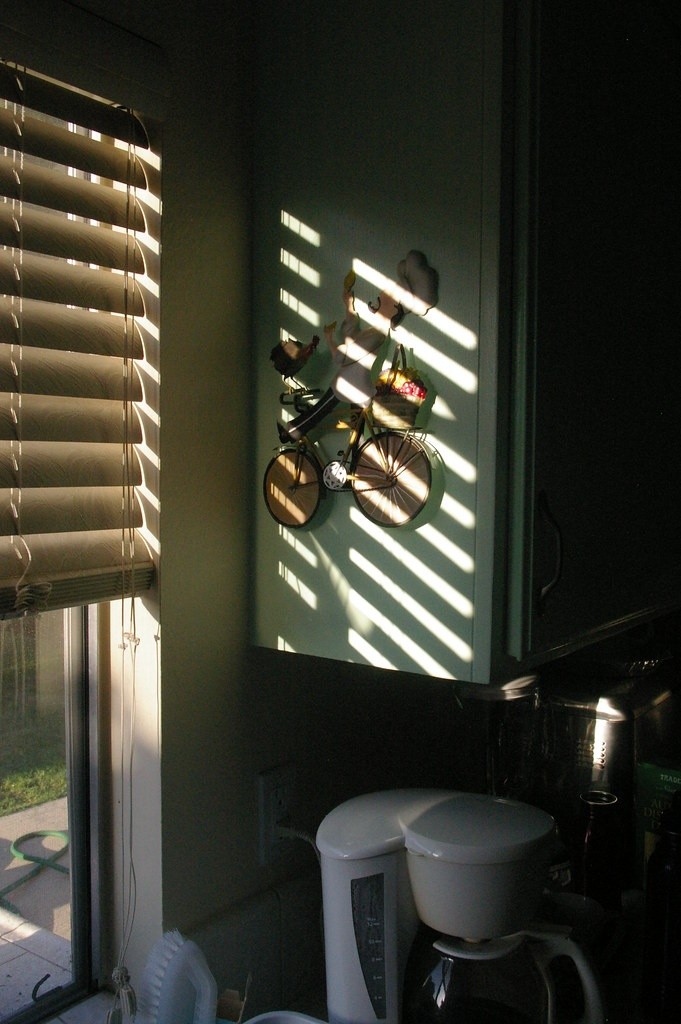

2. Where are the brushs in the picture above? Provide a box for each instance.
[134,925,220,1024]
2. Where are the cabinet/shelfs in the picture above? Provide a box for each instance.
[249,1,681,683]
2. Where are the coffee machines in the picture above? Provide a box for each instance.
[316,790,607,1023]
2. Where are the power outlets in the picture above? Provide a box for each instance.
[257,764,296,864]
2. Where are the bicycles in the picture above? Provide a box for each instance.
[261,386,433,528]
